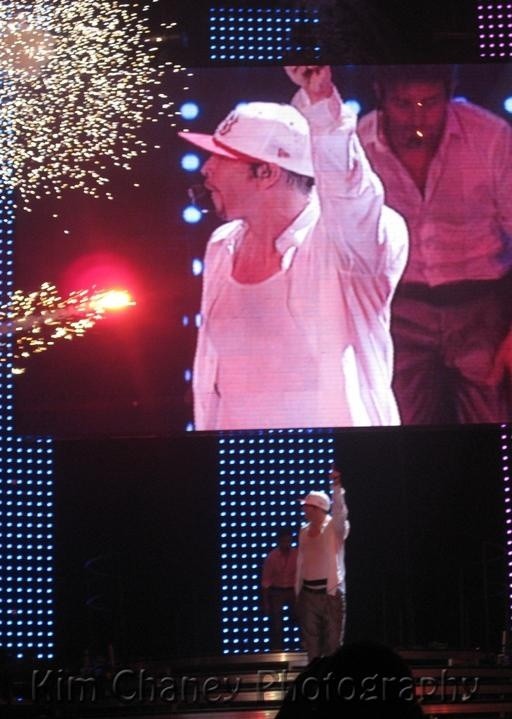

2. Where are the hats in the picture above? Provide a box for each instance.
[297,491,330,511]
[178,101,316,177]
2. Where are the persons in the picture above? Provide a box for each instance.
[177,65,410,430]
[293,462,352,665]
[275,641,427,718]
[260,528,308,653]
[1,637,123,718]
[357,65,511,426]
